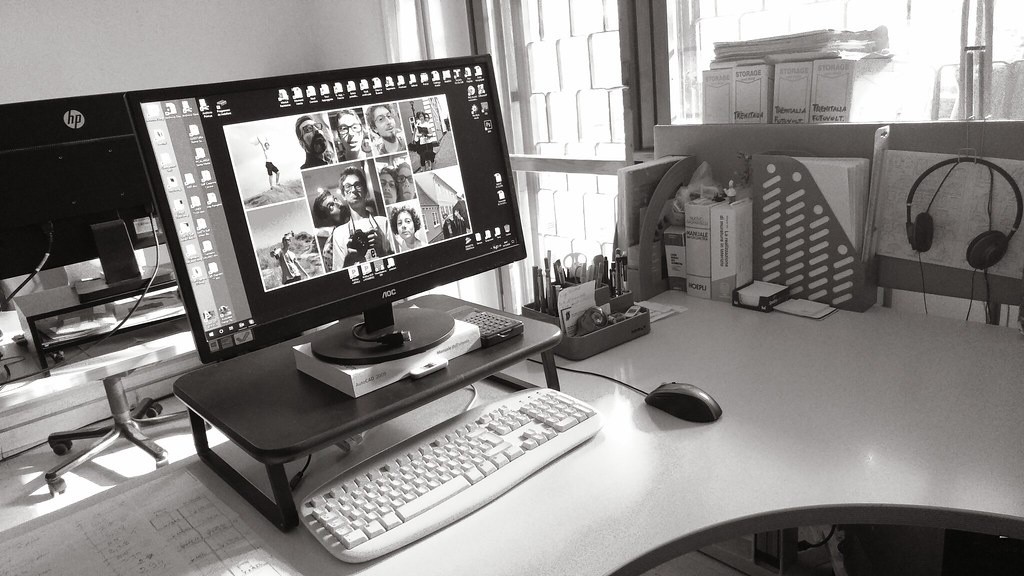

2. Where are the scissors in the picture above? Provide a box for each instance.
[562,253,587,280]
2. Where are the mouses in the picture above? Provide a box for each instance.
[644,381,723,423]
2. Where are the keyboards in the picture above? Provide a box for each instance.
[298,387,603,564]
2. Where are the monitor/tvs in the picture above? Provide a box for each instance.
[0,53,528,366]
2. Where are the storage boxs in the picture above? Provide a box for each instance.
[662,197,753,303]
[702,59,855,125]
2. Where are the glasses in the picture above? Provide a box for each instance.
[342,182,364,191]
[302,123,323,133]
[372,112,393,123]
[396,175,413,183]
[336,123,363,135]
[380,179,398,188]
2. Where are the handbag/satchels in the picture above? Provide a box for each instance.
[408,141,418,152]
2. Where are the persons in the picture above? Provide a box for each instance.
[256,104,470,286]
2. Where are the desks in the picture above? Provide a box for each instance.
[0,264,1024,575]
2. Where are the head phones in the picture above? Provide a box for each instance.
[906,157,1023,270]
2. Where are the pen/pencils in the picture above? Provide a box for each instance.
[534,249,630,316]
